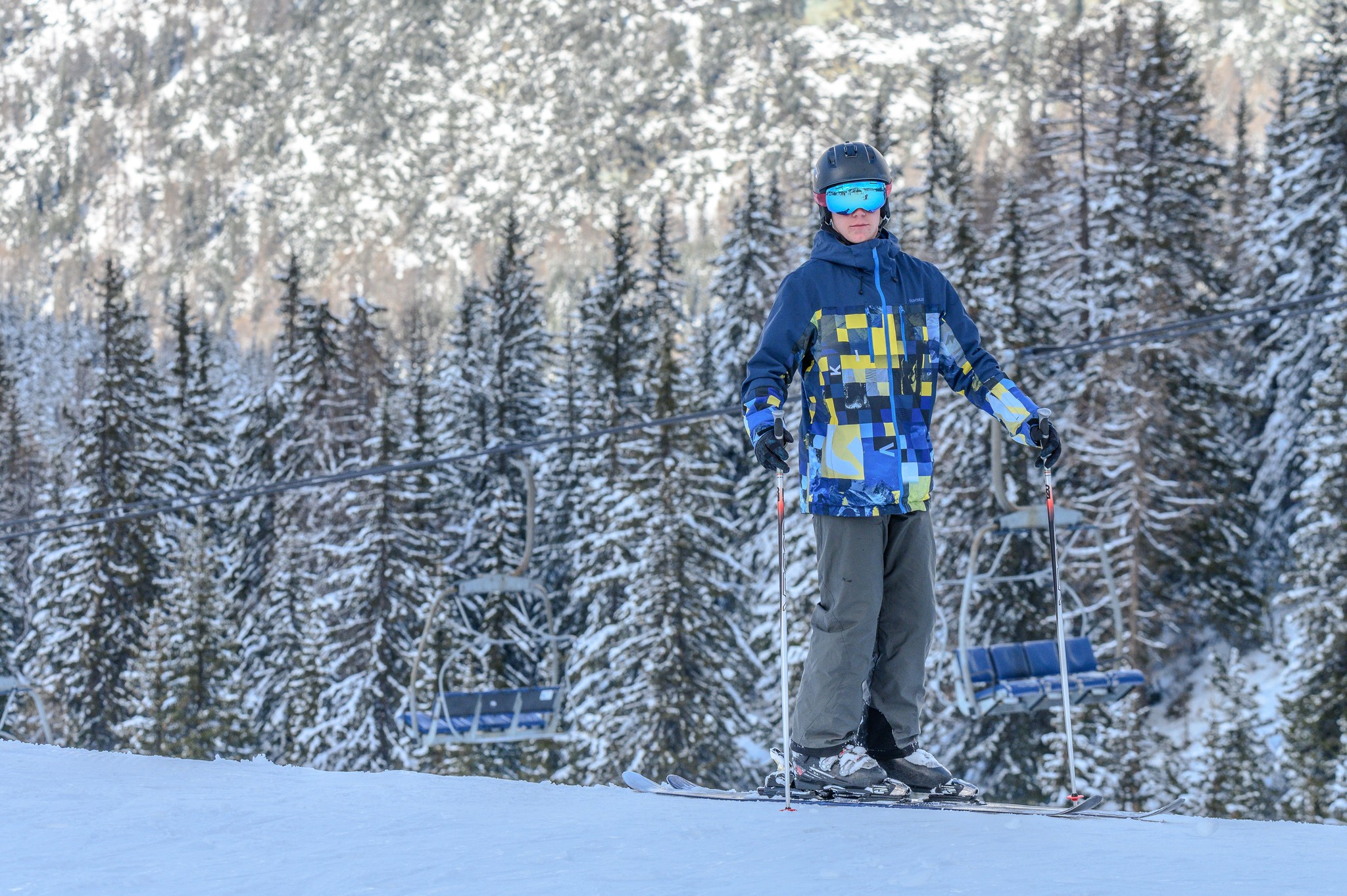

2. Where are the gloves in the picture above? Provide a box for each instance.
[754,428,794,474]
[1030,424,1061,469]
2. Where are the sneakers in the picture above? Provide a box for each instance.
[793,742,888,793]
[855,741,952,792]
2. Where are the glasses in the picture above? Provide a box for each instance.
[825,181,886,215]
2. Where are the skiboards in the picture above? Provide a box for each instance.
[620,769,1185,820]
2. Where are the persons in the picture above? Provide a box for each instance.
[737,142,1059,801]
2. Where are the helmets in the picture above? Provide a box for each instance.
[813,141,892,220]
